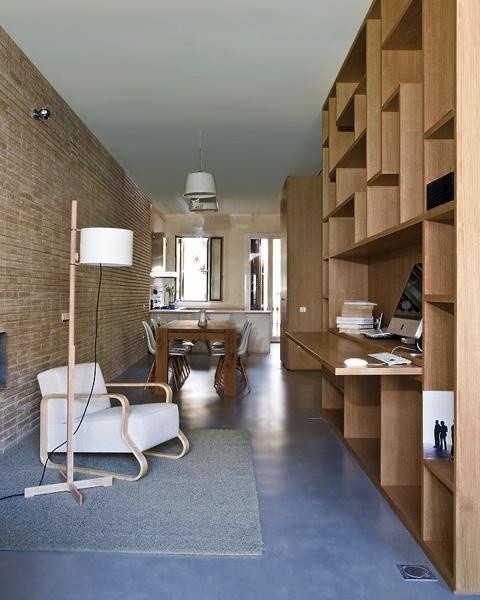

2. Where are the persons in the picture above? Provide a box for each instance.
[439,421,448,452]
[433,421,440,448]
[451,420,454,456]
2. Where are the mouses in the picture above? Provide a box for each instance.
[344,358,368,367]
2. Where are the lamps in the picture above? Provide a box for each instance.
[24,198,133,504]
[189,145,218,212]
[183,130,217,199]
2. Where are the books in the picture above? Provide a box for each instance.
[336,300,378,335]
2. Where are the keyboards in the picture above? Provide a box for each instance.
[368,352,412,367]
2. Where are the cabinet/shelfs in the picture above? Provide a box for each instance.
[279,176,322,371]
[285,0,480,562]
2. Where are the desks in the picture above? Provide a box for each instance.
[155,319,237,396]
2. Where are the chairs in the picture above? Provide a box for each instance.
[212,320,250,379]
[142,321,181,392]
[151,318,187,384]
[36,360,190,483]
[210,320,253,388]
[209,323,254,393]
[158,316,190,375]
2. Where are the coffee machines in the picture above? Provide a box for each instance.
[151,279,170,310]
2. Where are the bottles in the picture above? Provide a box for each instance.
[199,309,207,326]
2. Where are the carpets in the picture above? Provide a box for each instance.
[1,425,264,556]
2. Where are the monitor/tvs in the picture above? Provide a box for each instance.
[385,262,422,345]
